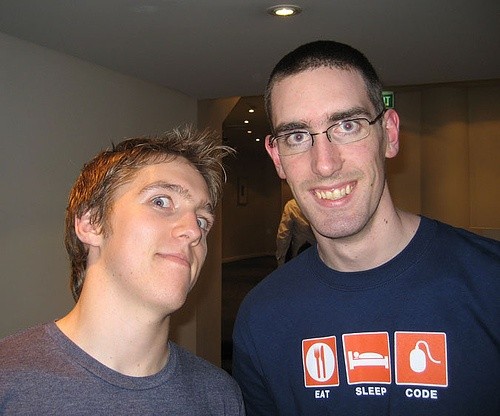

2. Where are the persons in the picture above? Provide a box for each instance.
[0,118,249,416]
[230,39,500,416]
[275,199,316,270]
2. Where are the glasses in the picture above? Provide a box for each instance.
[267,105,388,157]
